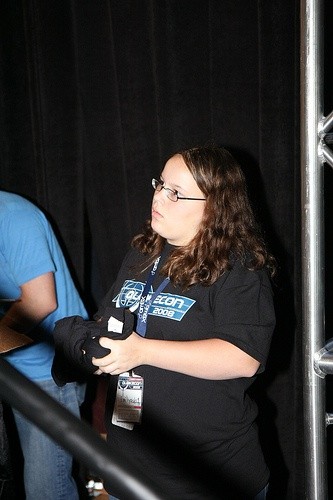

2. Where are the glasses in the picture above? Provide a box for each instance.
[152,178,206,202]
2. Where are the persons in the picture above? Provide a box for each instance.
[0,190,90,500]
[92,143,277,500]
